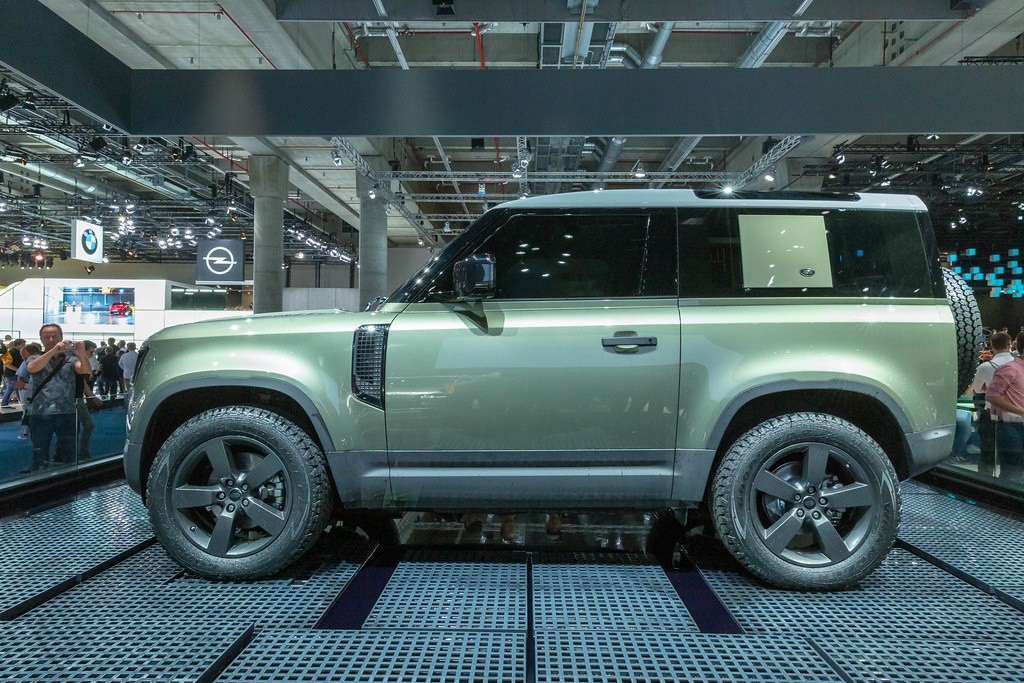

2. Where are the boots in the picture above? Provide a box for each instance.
[20,447,41,472]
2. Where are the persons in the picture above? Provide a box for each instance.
[0,334,139,465]
[25,324,91,470]
[971,332,1024,486]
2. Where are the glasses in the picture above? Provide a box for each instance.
[88,350,94,355]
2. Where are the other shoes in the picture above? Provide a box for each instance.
[1,403,16,409]
[76,453,91,463]
[50,454,65,466]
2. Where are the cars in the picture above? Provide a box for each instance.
[110,301,132,315]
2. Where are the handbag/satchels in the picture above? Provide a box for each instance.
[21,407,28,426]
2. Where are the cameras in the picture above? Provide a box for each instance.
[64,341,77,349]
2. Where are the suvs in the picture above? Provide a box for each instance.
[123,187,984,594]
